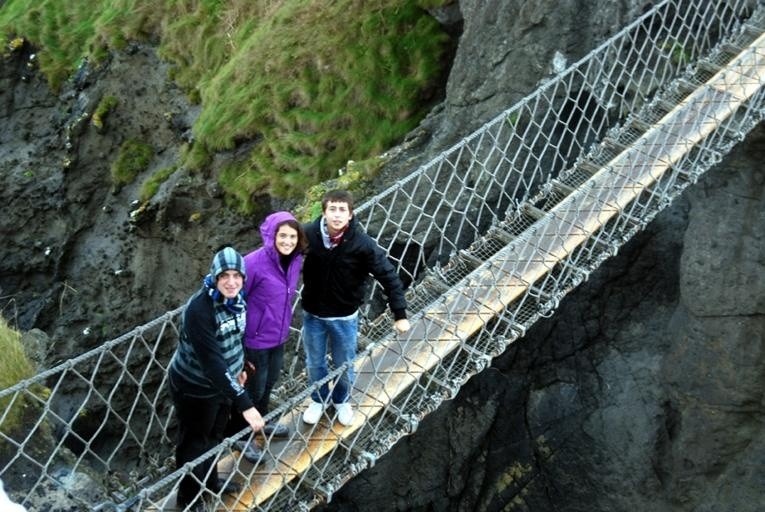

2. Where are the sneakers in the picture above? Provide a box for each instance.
[303,398,333,424]
[233,439,267,463]
[331,399,354,428]
[257,421,289,437]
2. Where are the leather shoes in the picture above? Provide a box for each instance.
[210,478,241,493]
[190,498,209,512]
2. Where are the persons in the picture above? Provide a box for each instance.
[167,247,265,512]
[302,191,412,426]
[233,211,309,464]
